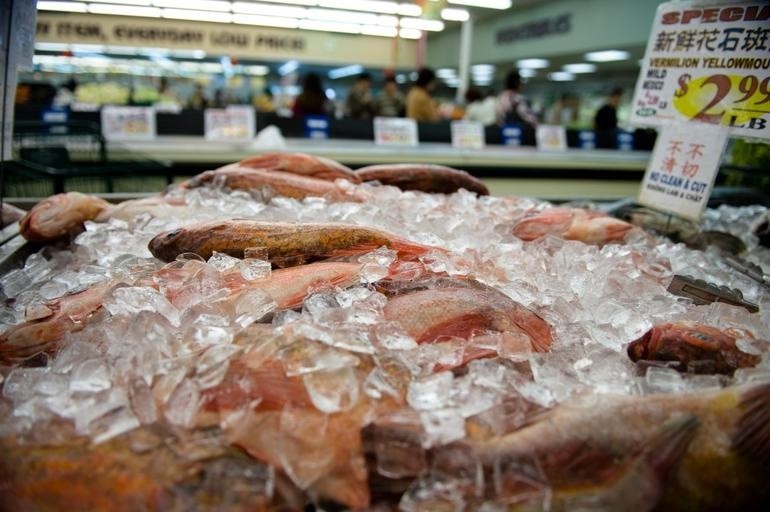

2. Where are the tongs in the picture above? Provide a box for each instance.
[667,247,770,313]
